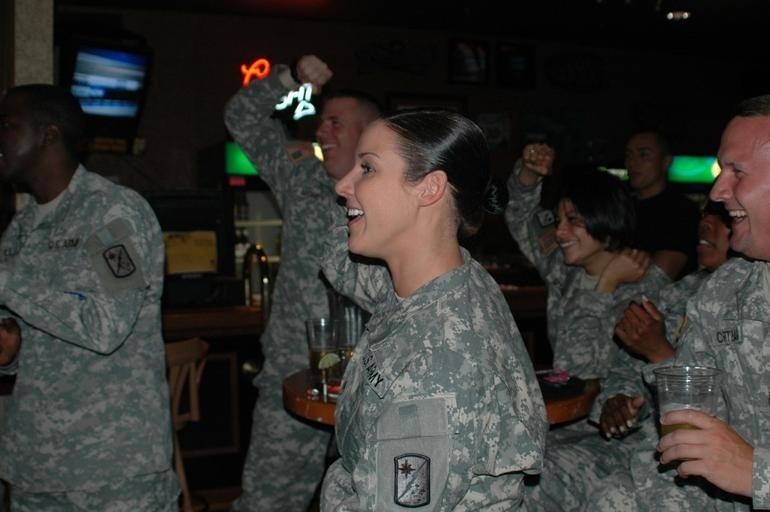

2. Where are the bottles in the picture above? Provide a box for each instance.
[245,244,270,309]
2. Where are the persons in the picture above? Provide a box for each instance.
[319,107,550,512]
[505,97,769,512]
[224,54,387,512]
[0,83,183,512]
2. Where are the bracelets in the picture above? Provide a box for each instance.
[290,65,302,85]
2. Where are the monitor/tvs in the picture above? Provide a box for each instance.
[57,41,153,140]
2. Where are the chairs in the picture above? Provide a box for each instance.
[159,337,211,511]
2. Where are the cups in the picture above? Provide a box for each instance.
[656,367,722,465]
[305,316,340,405]
[337,308,364,388]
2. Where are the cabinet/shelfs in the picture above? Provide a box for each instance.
[234,217,286,263]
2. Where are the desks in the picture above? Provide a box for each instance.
[283,364,598,511]
